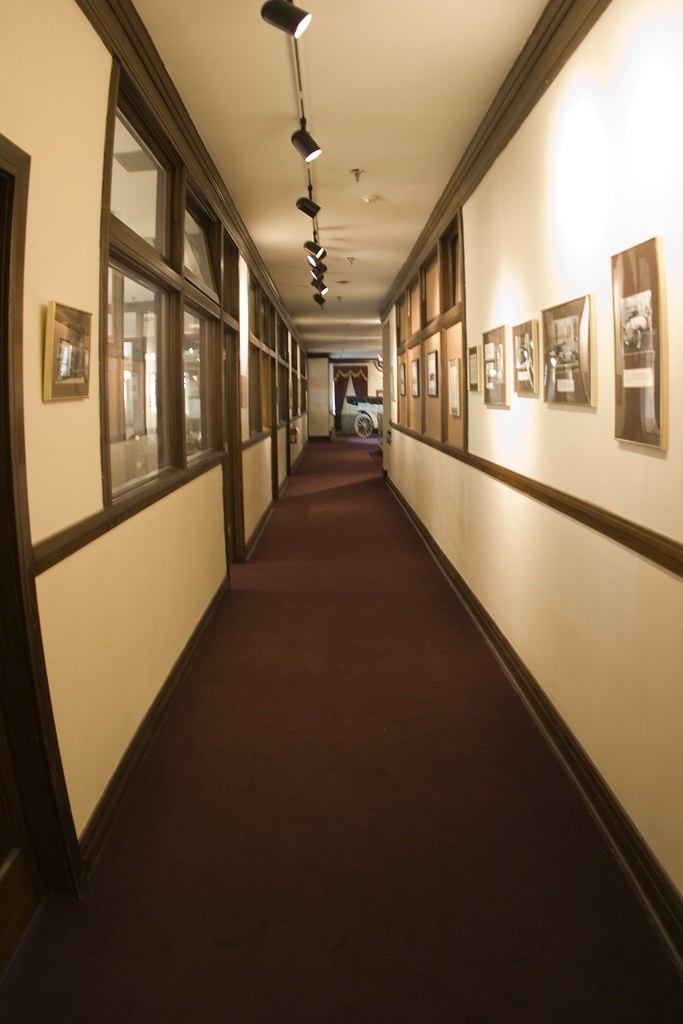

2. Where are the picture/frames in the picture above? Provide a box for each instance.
[511,319,540,394]
[540,293,597,408]
[609,238,670,453]
[410,359,419,397]
[399,363,406,396]
[426,350,437,397]
[446,358,462,419]
[42,300,93,402]
[480,325,509,407]
[467,346,482,393]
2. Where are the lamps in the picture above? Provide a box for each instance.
[295,186,321,219]
[260,0,312,39]
[291,119,322,163]
[303,234,328,305]
